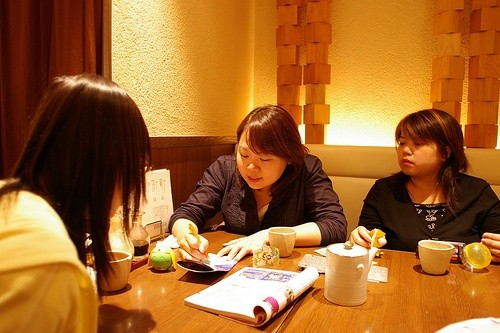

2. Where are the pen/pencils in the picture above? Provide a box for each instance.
[187,223,210,261]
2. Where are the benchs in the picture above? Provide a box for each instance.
[303,144,500,241]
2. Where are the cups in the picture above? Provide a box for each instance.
[94,250,132,292]
[268,227,296,258]
[418,240,454,275]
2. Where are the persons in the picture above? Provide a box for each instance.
[343,108,499,265]
[167,104,348,266]
[0,72,152,333]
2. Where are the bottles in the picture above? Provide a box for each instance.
[128,210,150,262]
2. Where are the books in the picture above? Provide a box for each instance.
[184,265,321,329]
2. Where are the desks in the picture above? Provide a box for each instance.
[99,232,499,333]
